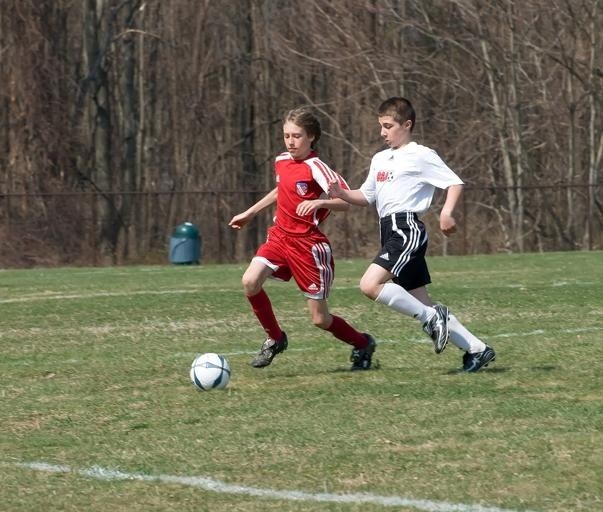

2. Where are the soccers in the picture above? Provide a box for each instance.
[189,353,230,392]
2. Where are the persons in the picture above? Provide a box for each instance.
[326,96,496,376]
[227,107,376,372]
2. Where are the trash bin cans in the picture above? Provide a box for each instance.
[169,222,202,265]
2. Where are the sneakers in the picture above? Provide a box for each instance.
[422,305,450,354]
[350,332,375,370]
[463,343,496,373]
[252,331,288,368]
